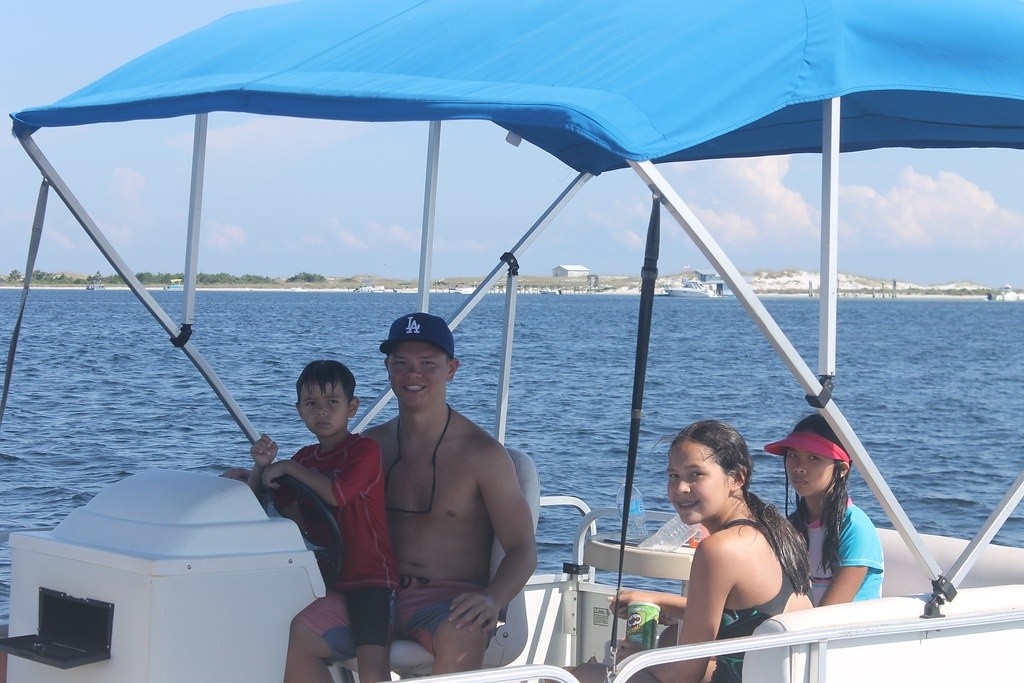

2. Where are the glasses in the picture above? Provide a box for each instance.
[381,455,436,513]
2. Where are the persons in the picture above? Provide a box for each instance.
[570,417,816,683]
[223,312,537,683]
[764,411,884,608]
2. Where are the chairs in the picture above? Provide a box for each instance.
[338,450,540,674]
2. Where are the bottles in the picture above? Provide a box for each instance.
[616,479,648,543]
[638,515,700,552]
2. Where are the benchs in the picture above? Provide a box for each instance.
[741,527,1024,683]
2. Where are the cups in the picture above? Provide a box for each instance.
[625,603,661,651]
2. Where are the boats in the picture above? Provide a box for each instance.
[664,280,718,298]
[0,0,1022,681]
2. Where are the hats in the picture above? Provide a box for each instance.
[764,433,850,464]
[380,312,454,359]
